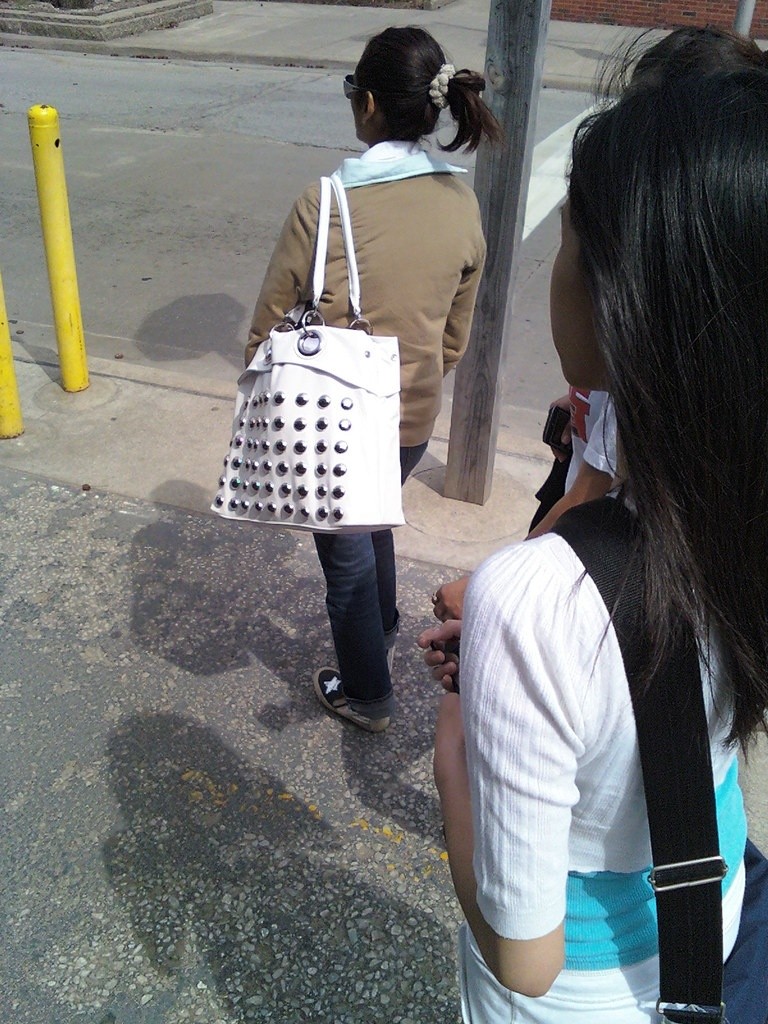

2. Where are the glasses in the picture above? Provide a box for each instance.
[343,74,377,100]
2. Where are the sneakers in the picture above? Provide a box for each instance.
[385,643,397,677]
[314,667,391,732]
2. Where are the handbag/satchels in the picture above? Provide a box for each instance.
[209,173,404,534]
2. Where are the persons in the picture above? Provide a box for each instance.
[433,13,768,1024]
[245,25,487,733]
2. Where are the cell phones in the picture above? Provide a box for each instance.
[542,405,572,456]
